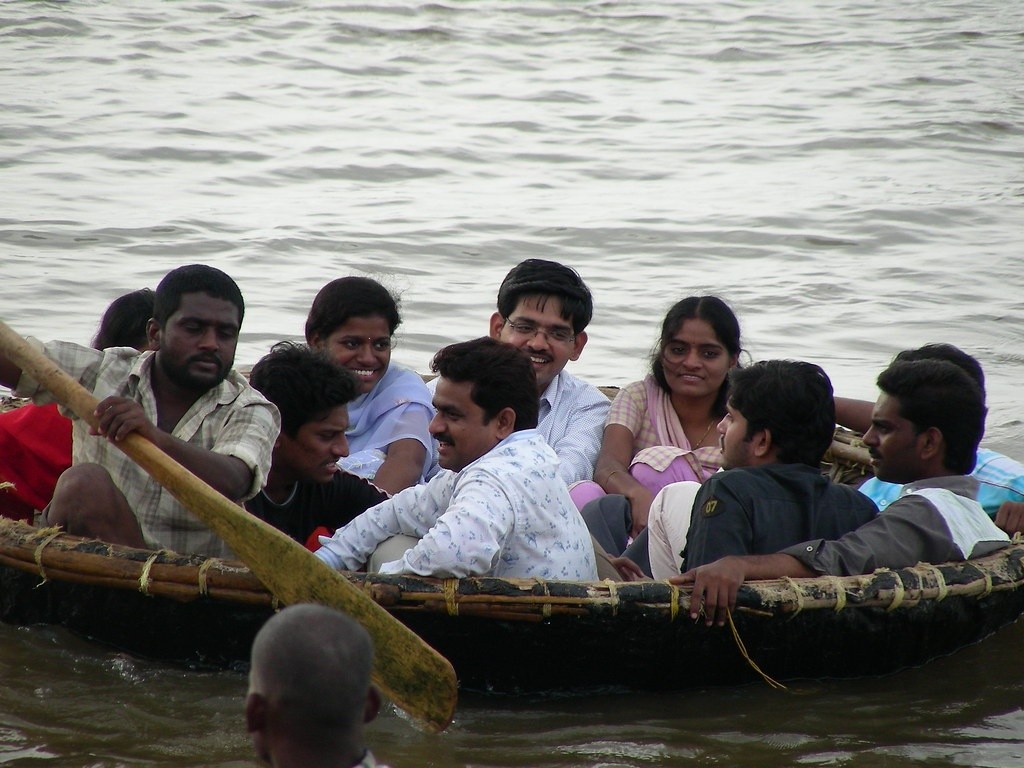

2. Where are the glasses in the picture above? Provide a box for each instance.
[506,318,575,344]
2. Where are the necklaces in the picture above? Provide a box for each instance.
[693,418,717,450]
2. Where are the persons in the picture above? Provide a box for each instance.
[0,276,1024,624]
[245,604,387,768]
[0,266,280,566]
[422,258,611,487]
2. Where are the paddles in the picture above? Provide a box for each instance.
[0,317,457,735]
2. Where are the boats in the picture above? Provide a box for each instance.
[0,386,1024,690]
